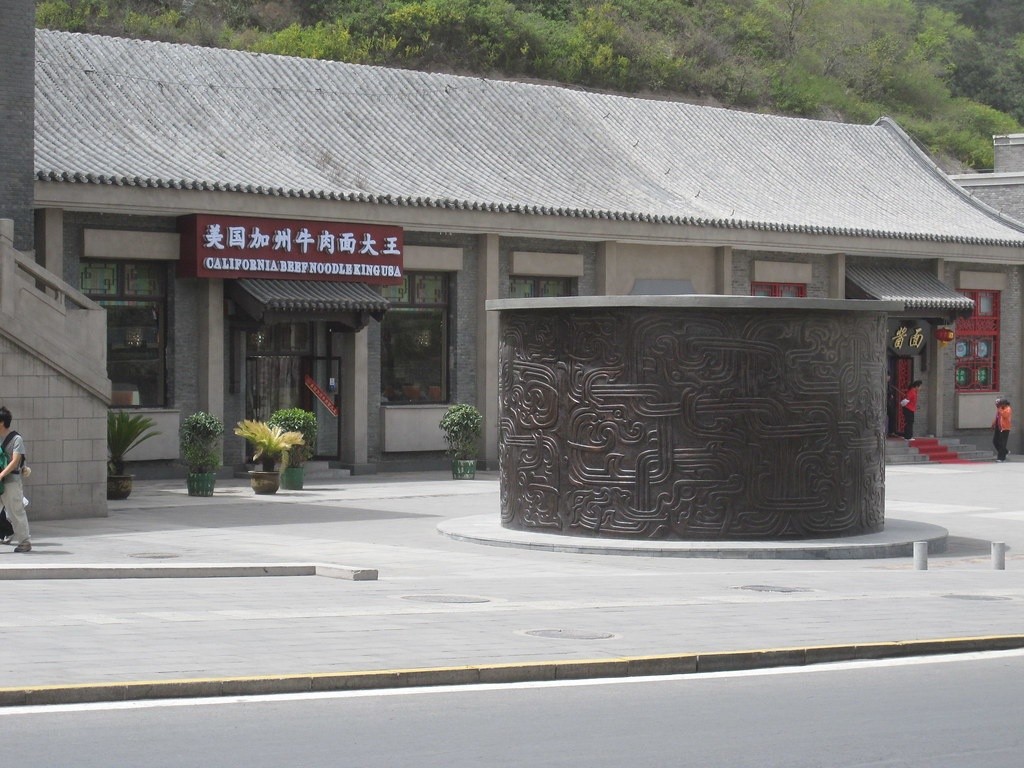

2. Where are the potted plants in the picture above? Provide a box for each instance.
[234,419,305,494]
[439,404,482,480]
[180,411,224,496]
[267,407,320,491]
[106,408,163,500]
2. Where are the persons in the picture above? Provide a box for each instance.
[900,380,922,441]
[887,372,898,437]
[0,407,32,553]
[991,399,1011,462]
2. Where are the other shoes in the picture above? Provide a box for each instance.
[996,459,1006,462]
[14,544,32,553]
[905,438,915,441]
[1006,450,1012,458]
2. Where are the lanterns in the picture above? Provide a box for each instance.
[936,329,954,346]
